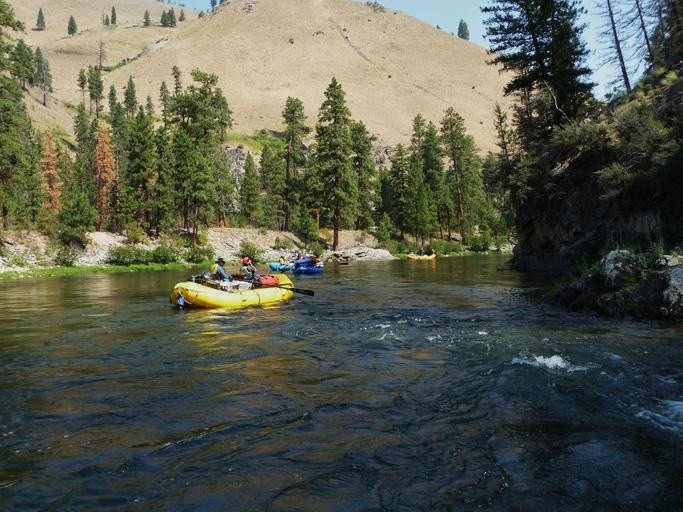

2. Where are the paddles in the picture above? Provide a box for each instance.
[251,280,315,296]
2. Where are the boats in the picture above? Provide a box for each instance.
[406,253,435,260]
[268,258,326,275]
[168,272,295,311]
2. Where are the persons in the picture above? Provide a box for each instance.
[240,255,259,280]
[209,258,230,280]
[278,251,316,266]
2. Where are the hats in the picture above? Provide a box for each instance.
[243,256,251,265]
[215,258,224,263]
